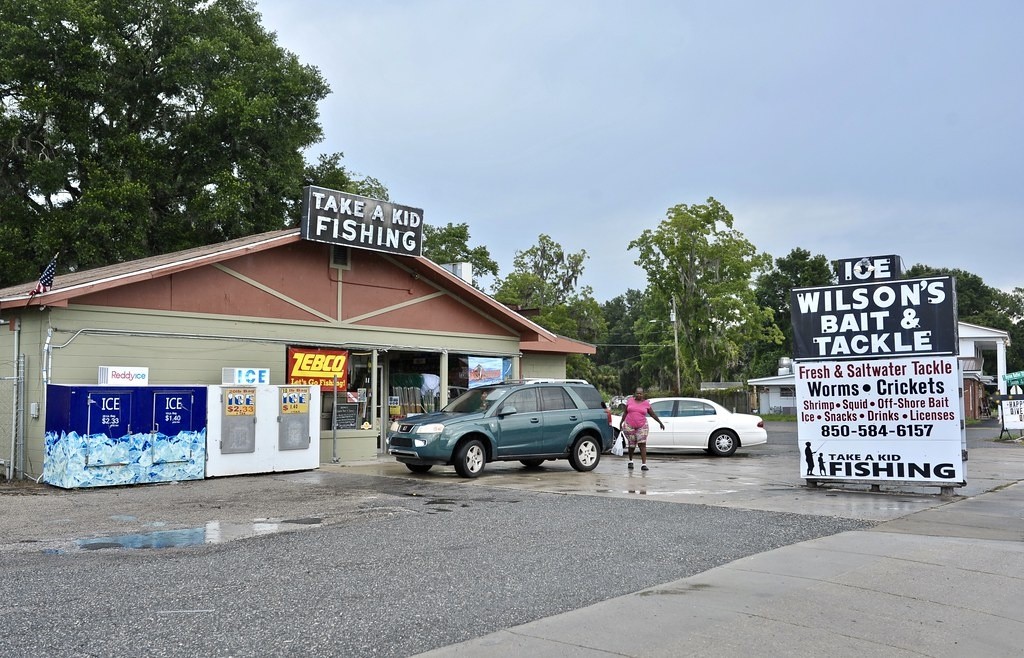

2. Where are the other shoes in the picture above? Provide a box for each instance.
[628,463,634,469]
[641,466,649,470]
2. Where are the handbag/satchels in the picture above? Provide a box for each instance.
[611,431,624,456]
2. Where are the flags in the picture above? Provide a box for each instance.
[29,255,56,295]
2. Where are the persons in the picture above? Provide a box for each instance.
[350,368,368,391]
[621,387,665,470]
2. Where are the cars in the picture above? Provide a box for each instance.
[609,397,768,457]
[611,394,633,410]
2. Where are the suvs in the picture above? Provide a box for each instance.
[386,381,615,479]
[496,378,589,412]
